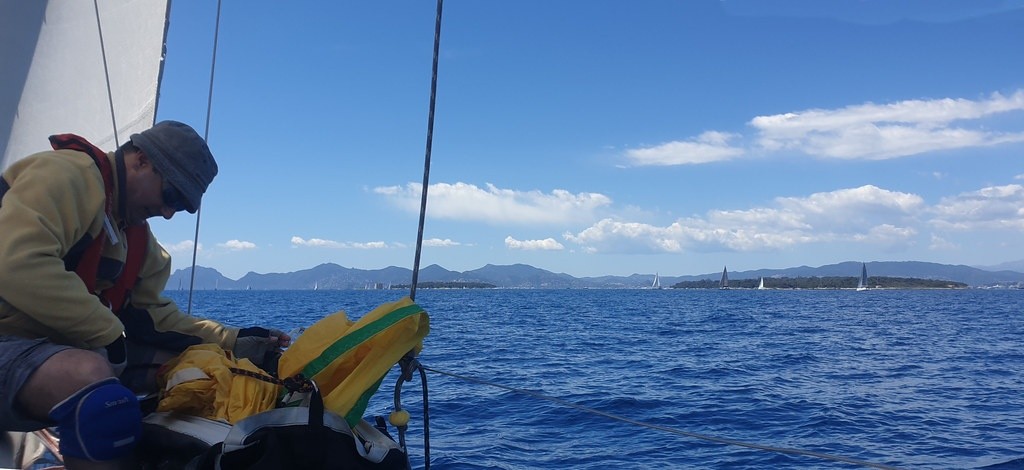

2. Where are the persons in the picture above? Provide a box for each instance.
[0,118,291,470]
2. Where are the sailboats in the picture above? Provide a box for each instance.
[857,261,869,291]
[718,266,731,290]
[651,273,662,290]
[0,0,444,469]
[757,277,764,290]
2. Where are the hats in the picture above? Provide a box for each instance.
[132,120,218,209]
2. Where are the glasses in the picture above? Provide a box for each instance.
[162,186,184,211]
